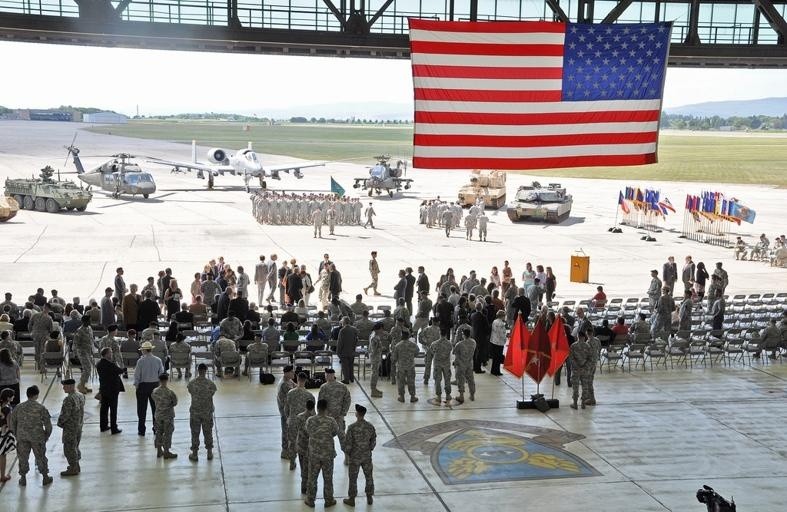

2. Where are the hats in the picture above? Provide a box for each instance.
[61,379,75,385]
[356,404,366,412]
[284,365,293,373]
[138,342,155,351]
[324,368,335,373]
[106,287,113,292]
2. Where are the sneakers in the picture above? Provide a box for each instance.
[1,475,10,481]
[266,298,270,303]
[271,300,276,302]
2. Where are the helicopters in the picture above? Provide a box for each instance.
[324,151,414,198]
[53,131,165,201]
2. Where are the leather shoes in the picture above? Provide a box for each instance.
[476,370,485,373]
[491,371,502,376]
[138,431,145,436]
[342,378,354,384]
[101,427,110,432]
[111,429,121,434]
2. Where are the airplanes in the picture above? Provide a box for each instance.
[138,137,327,193]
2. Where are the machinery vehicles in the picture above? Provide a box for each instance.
[457,169,510,211]
[505,181,573,224]
[3,165,92,214]
[0,185,19,225]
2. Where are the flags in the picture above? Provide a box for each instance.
[406,18,674,169]
[331,176,345,197]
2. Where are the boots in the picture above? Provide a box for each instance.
[157,447,212,461]
[374,289,381,295]
[768,351,776,359]
[77,382,91,394]
[570,398,596,409]
[371,378,474,403]
[242,367,247,375]
[753,351,760,358]
[176,369,182,378]
[281,450,296,470]
[363,288,369,295]
[19,461,80,485]
[95,394,101,399]
[185,369,192,378]
[304,495,373,508]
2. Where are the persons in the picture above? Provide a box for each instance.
[1,190,786,507]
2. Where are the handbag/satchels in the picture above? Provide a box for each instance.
[306,284,314,293]
[260,374,274,385]
